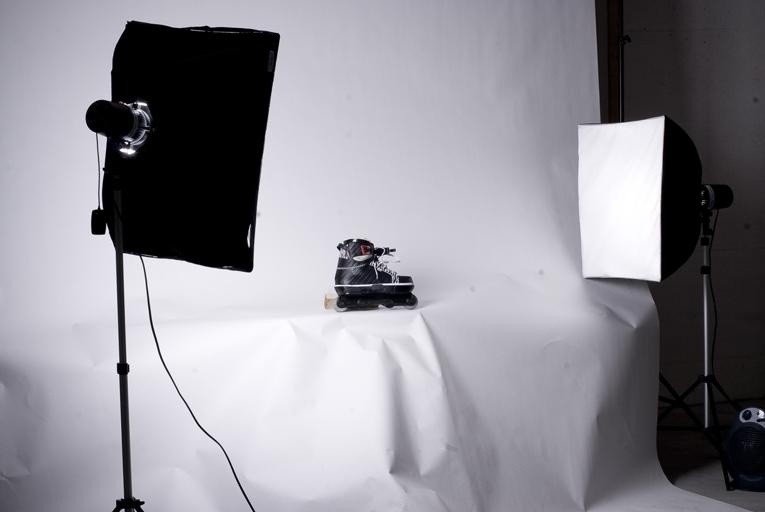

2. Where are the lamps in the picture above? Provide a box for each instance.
[82,19,280,512]
[577,114,734,461]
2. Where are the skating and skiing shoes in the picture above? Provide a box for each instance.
[334,239,418,312]
